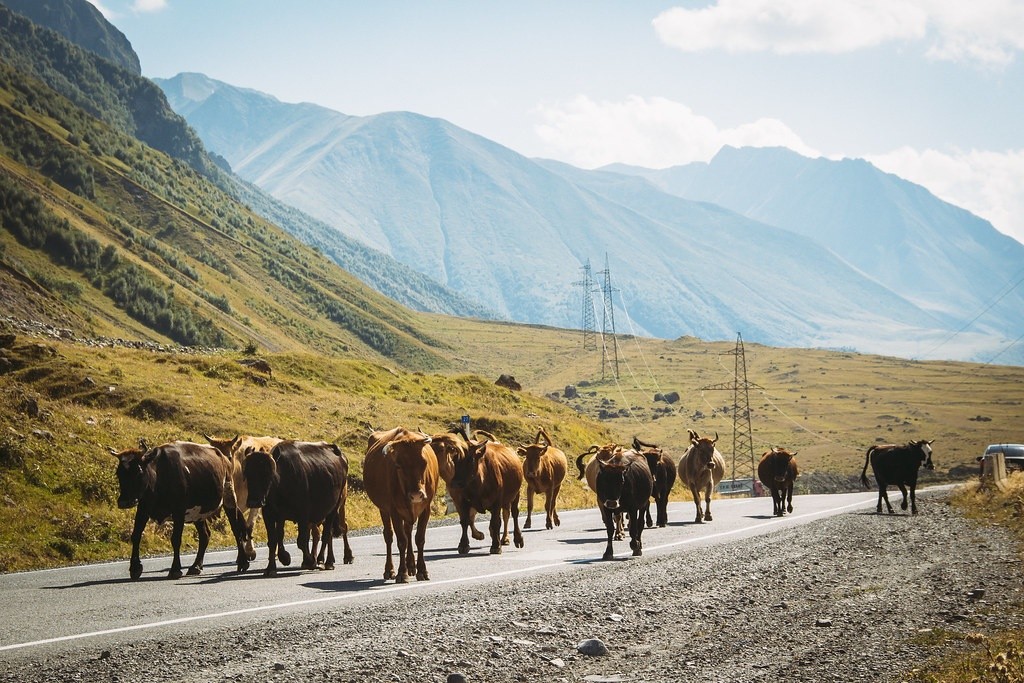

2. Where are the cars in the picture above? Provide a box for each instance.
[976,443,1024,483]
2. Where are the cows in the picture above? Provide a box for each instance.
[107,425,936,583]
